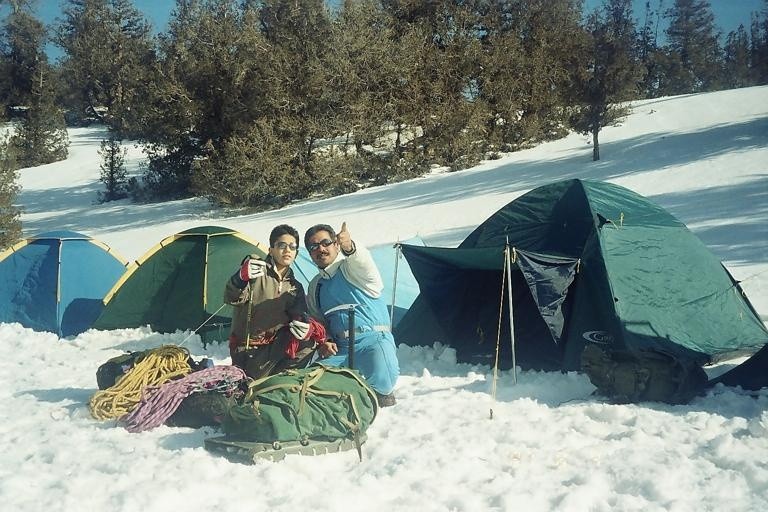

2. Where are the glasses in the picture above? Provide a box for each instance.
[274,242,299,251]
[308,239,336,254]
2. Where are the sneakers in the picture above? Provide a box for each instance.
[375,392,397,407]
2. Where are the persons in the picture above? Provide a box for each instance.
[223,225,326,382]
[303,221,400,408]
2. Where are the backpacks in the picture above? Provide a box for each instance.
[580,345,708,404]
[96,348,380,442]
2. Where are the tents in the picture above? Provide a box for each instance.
[290,233,431,340]
[90,221,278,349]
[388,176,768,406]
[0,225,131,342]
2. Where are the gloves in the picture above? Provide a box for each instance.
[240,253,266,282]
[289,316,314,342]
